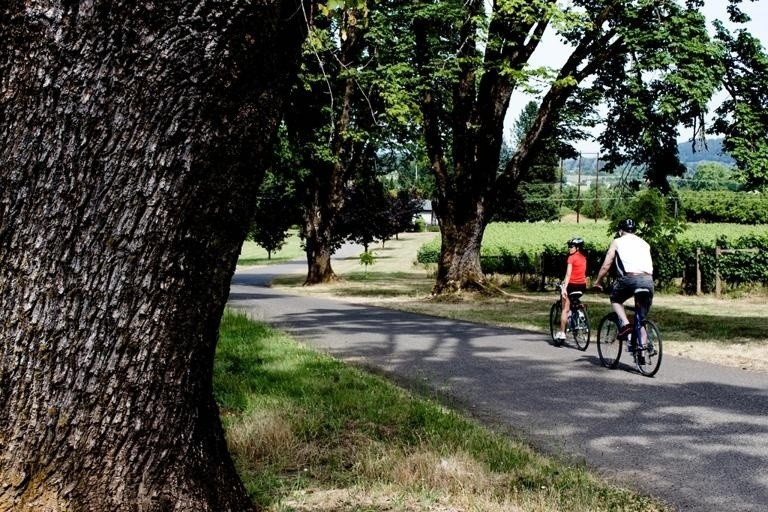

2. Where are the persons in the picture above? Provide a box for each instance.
[552,237,587,341]
[592,218,654,365]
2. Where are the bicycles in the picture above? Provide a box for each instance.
[591,285,662,377]
[549,282,591,351]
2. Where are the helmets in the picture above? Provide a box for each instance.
[618,218,636,229]
[567,238,584,244]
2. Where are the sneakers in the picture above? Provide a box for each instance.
[554,332,567,340]
[617,323,633,339]
